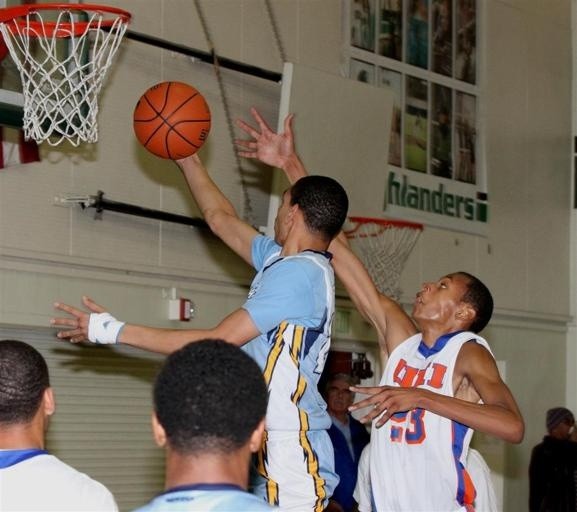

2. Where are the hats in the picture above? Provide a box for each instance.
[547,407,574,431]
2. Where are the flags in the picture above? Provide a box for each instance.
[0,1,42,168]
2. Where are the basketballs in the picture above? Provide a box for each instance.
[134,82,211,160]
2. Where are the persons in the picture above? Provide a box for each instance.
[352,352,374,382]
[349,0,476,183]
[127,336,291,510]
[0,337,120,512]
[48,150,358,510]
[230,103,525,511]
[526,407,575,510]
[317,370,371,512]
